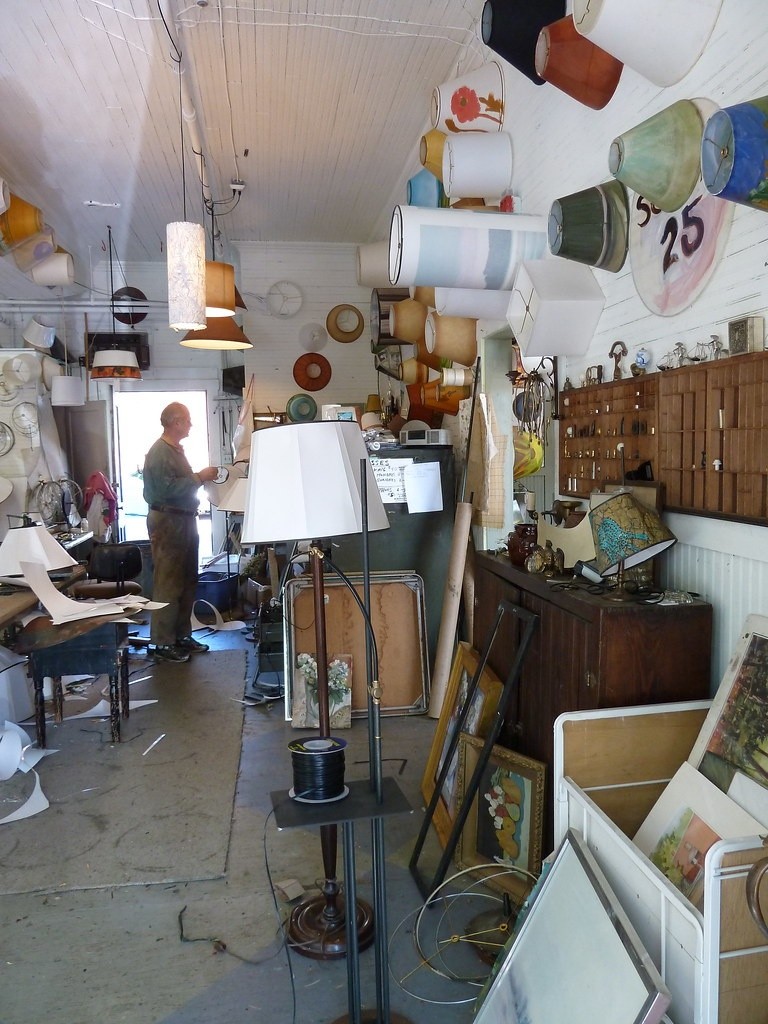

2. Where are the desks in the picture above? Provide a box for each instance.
[28,621,129,752]
[0,565,86,628]
[281,569,429,722]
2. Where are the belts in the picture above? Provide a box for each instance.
[150,504,199,518]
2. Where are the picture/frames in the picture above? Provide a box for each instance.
[408,600,538,909]
[455,729,545,907]
[421,641,505,850]
[631,613,768,913]
[468,829,671,1024]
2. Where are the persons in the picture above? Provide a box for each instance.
[143,401,219,663]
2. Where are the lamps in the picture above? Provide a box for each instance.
[241,419,390,960]
[585,491,676,601]
[0,514,80,620]
[0,0,768,520]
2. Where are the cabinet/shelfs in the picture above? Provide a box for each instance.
[554,698,768,1024]
[556,351,768,527]
[474,551,712,861]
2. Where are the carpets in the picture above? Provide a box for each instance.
[0,649,249,895]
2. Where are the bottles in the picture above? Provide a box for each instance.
[81,518,89,532]
[563,511,587,529]
[507,524,538,566]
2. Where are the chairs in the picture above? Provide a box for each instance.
[75,545,141,600]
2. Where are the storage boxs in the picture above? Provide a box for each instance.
[194,570,239,614]
[728,316,763,357]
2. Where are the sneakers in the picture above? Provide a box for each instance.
[154,645,191,662]
[177,636,209,653]
